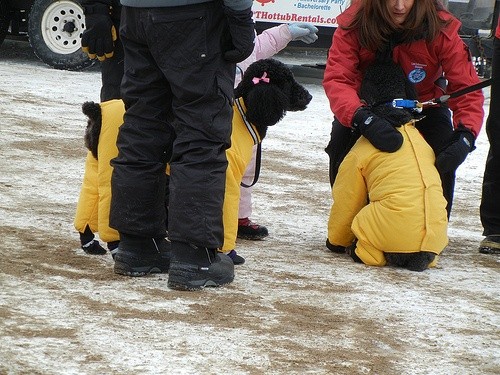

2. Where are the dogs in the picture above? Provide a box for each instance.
[74,58,313,264]
[326,56,448,271]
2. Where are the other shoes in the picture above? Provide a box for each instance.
[237,221,269,240]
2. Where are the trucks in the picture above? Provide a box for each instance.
[0,1,354,72]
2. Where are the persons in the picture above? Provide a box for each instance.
[475,0,500,255]
[85,0,318,290]
[322,0,485,221]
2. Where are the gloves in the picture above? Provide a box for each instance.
[223,7,256,63]
[81,2,117,61]
[288,21,319,44]
[434,131,476,171]
[353,106,403,153]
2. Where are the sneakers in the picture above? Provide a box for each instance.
[115,232,170,276]
[479,238,500,253]
[168,240,233,291]
